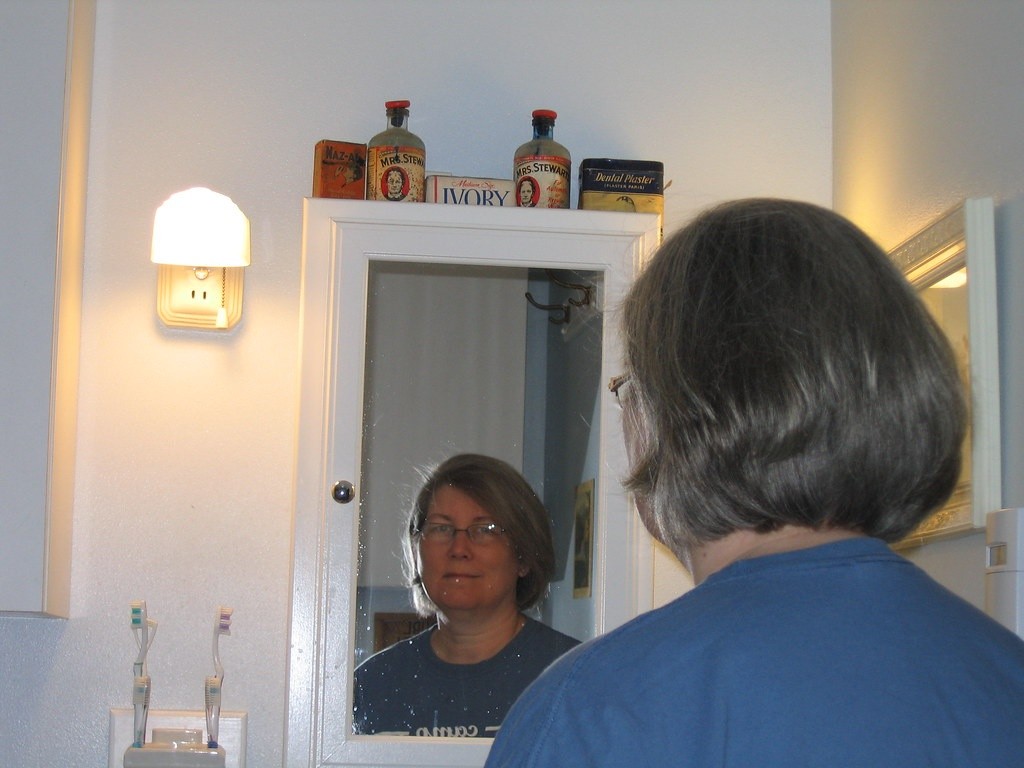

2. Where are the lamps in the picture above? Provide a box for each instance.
[151,187,251,330]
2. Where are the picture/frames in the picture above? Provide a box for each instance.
[852,196,1002,550]
[573,479,594,599]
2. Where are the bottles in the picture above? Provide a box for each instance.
[366,101,425,202]
[514,110,571,209]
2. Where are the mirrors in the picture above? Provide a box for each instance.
[282,198,661,768]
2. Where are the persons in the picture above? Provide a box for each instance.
[484,196,1023,768]
[354,455,584,738]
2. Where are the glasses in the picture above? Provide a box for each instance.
[413,521,502,547]
[608,369,637,410]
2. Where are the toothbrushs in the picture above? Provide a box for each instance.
[205,609,232,749]
[130,602,151,747]
